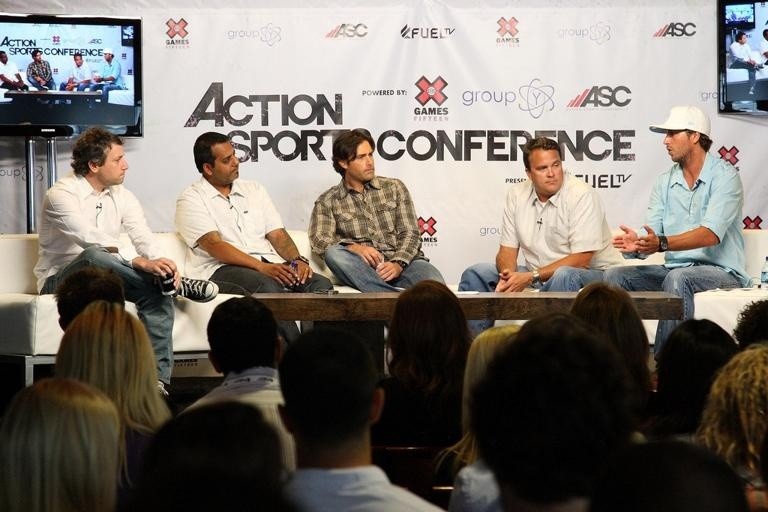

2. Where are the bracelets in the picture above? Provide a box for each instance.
[397,260,407,268]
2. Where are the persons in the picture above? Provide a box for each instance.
[59,52,92,105]
[1,268,767,512]
[760,28,768,66]
[26,50,56,105]
[90,48,124,108]
[175,132,333,344]
[0,51,29,91]
[458,138,612,339]
[33,128,220,396]
[729,31,764,95]
[602,105,754,367]
[307,129,446,293]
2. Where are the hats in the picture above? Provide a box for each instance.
[648,106,712,137]
[102,48,115,57]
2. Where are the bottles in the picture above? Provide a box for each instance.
[286,261,298,291]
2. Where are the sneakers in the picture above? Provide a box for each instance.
[176,276,219,304]
[749,87,760,95]
[758,62,765,68]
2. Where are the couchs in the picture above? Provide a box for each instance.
[465,230,767,344]
[0,233,460,385]
[0,72,61,104]
[726,51,768,84]
[62,74,135,107]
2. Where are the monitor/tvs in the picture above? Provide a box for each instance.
[717,0,768,115]
[0,12,143,138]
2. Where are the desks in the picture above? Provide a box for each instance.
[4,90,102,111]
[252,292,683,370]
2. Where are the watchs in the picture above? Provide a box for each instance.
[659,235,668,253]
[295,256,310,265]
